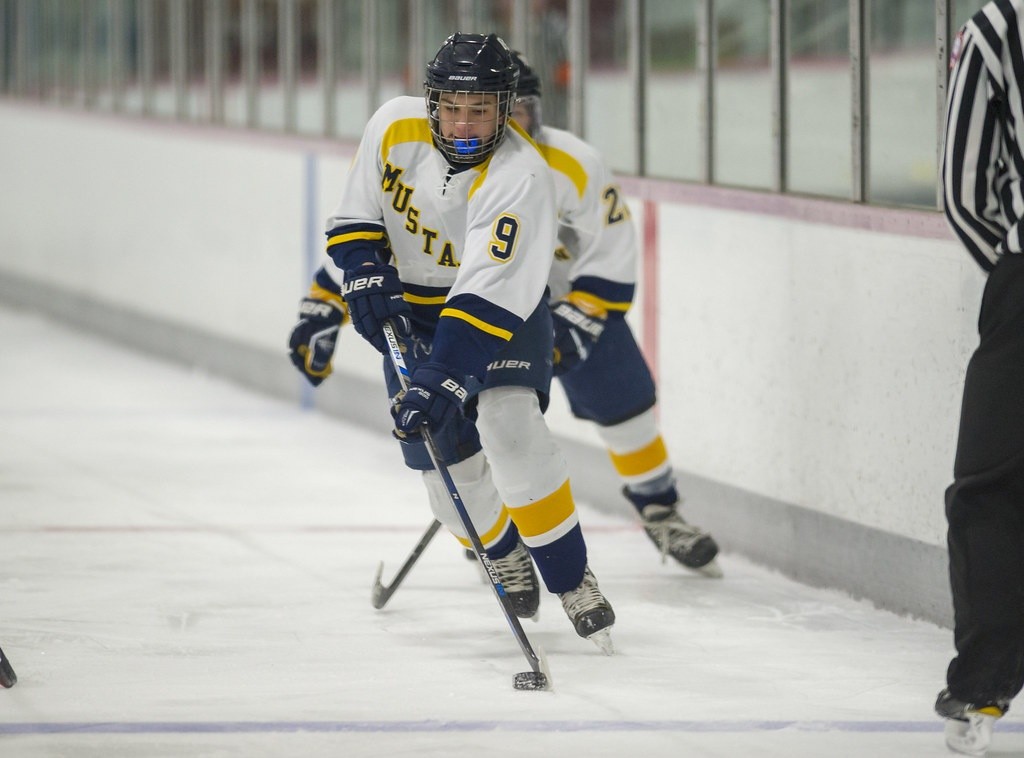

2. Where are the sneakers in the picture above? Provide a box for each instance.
[557,560,616,656]
[622,484,726,579]
[465,549,489,586]
[489,536,541,623]
[934,687,1010,758]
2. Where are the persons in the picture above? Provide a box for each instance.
[322,31,620,656]
[933,1,1024,756]
[288,47,728,576]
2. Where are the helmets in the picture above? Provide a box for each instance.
[510,49,542,139]
[423,31,520,169]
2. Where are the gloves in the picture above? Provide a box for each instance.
[390,362,478,444]
[549,300,607,377]
[288,297,344,386]
[341,263,413,357]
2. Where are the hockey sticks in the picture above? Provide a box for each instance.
[383,320,555,693]
[370,518,441,610]
[0,647,18,689]
